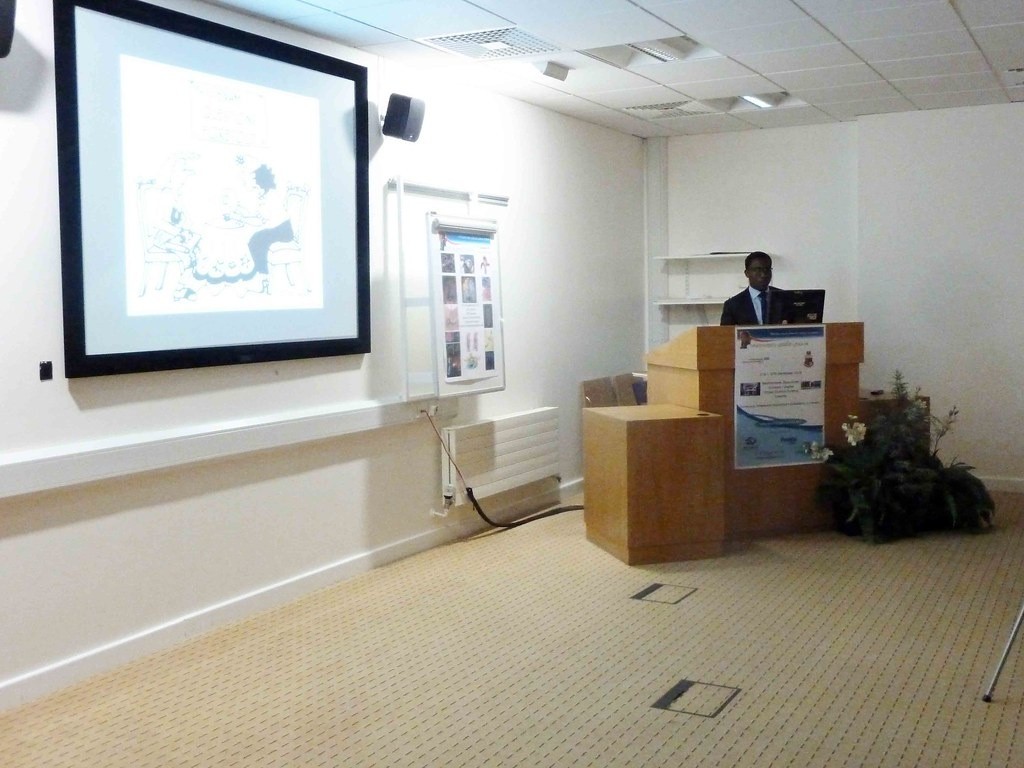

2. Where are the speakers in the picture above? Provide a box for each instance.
[0,0,16,57]
[380,91,426,140]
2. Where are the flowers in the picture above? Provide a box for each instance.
[801,368,996,544]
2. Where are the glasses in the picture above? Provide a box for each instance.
[746,267,773,274]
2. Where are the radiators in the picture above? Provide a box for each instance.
[430,406,563,519]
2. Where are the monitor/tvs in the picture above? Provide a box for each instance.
[763,288,825,326]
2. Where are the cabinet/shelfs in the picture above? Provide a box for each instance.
[654,256,754,304]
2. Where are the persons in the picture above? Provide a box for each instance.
[720,251,786,326]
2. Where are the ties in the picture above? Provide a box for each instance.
[758,291,768,325]
